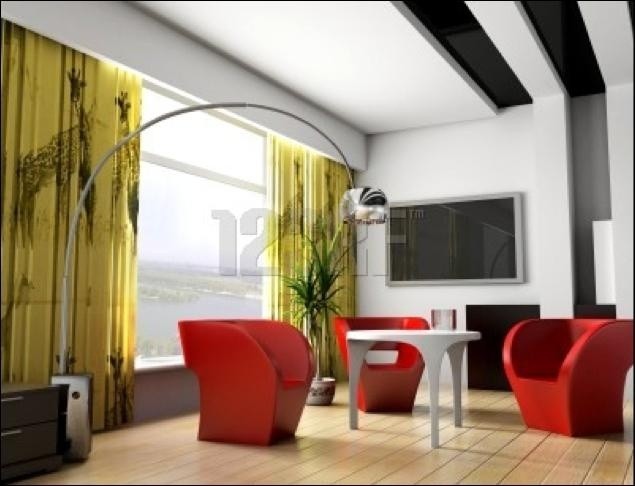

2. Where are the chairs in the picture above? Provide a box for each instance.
[501,315,634,439]
[176,313,320,448]
[334,314,433,415]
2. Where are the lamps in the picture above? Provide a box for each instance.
[56,95,391,469]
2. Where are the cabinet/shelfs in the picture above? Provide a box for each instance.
[0,384,68,486]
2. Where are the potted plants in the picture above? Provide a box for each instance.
[264,210,361,408]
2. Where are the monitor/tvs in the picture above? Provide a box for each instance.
[385,191,525,286]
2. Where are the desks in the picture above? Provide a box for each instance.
[340,328,483,451]
